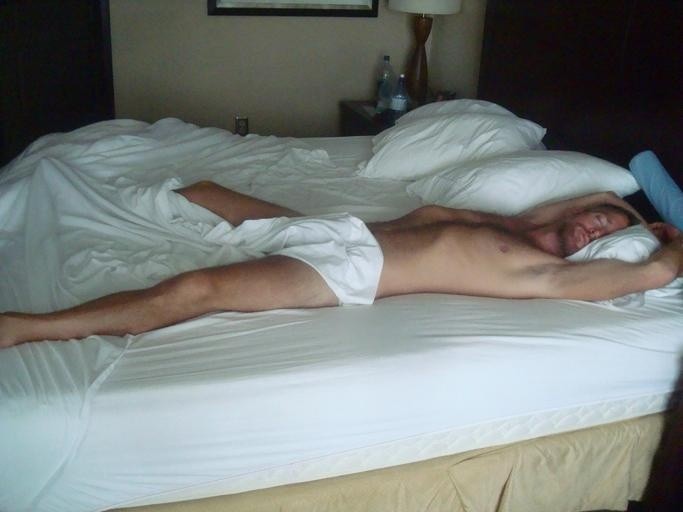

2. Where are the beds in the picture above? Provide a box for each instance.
[1,118,681,510]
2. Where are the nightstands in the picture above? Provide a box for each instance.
[337,101,410,135]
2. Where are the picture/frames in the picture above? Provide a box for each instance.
[206,0,380,17]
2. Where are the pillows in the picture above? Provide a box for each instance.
[384,99,516,118]
[408,147,641,215]
[353,116,542,179]
[564,224,680,305]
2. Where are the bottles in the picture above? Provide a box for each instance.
[388,74,408,119]
[375,55,392,103]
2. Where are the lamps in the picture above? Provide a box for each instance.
[385,0,461,102]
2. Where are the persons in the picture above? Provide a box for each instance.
[1,178,682,350]
